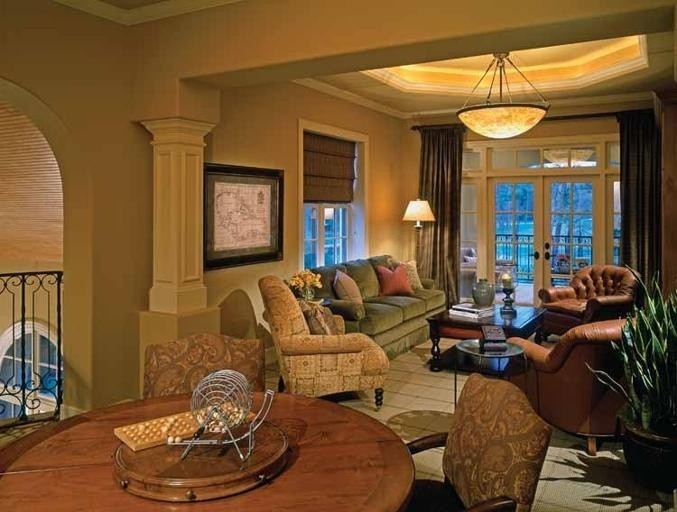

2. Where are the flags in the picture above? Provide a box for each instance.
[294,287,315,298]
[560,262,566,273]
[472,279,494,306]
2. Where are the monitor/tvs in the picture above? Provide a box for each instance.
[480,323,507,342]
[447,300,495,319]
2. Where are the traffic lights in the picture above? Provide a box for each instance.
[377,265,415,298]
[387,256,424,291]
[332,268,363,308]
[298,298,332,335]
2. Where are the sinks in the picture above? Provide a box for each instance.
[284,255,446,362]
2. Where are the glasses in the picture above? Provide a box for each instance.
[309,207,336,226]
[402,197,437,277]
[455,50,551,140]
[497,263,519,316]
[543,149,595,167]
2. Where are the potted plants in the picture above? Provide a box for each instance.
[203,161,284,271]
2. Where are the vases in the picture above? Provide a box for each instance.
[557,254,571,263]
[288,268,323,294]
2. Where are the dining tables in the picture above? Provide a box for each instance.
[585,262,677,490]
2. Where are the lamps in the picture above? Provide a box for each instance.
[461,247,501,300]
[507,317,638,456]
[399,373,552,512]
[143,333,265,399]
[259,274,390,409]
[535,263,641,345]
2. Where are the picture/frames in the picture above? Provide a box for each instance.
[453,339,541,421]
